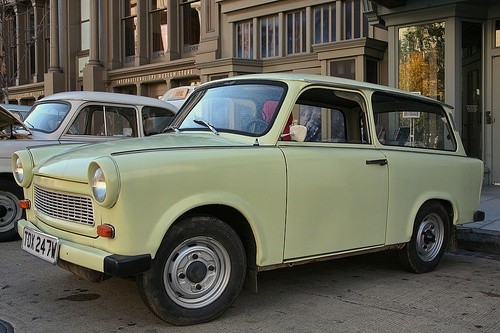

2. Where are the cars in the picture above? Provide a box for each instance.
[0,84,196,241]
[14,73,483,326]
